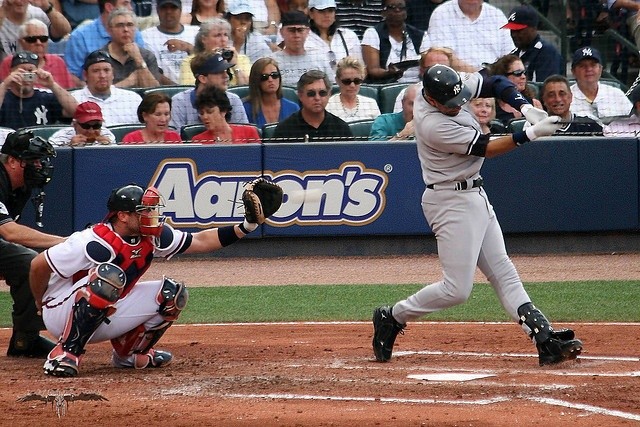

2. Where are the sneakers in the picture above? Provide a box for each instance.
[372,306,410,363]
[111,348,173,370]
[538,339,582,366]
[6,335,56,358]
[43,343,86,377]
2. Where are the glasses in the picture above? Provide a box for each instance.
[110,22,134,28]
[287,27,309,33]
[23,36,48,43]
[508,71,526,77]
[87,51,110,59]
[260,72,280,82]
[340,78,363,86]
[13,53,38,60]
[308,91,329,97]
[382,5,406,12]
[77,123,103,130]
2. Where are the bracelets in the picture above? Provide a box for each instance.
[44,5,54,12]
[233,68,241,74]
[217,223,241,248]
[395,132,402,140]
[511,131,530,147]
[507,91,529,111]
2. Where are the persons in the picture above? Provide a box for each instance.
[466,96,504,134]
[372,62,582,365]
[0,1,71,56]
[597,8,631,79]
[391,47,454,113]
[253,11,334,94]
[609,0,640,120]
[325,57,382,126]
[0,128,73,359]
[68,49,142,129]
[602,82,640,136]
[226,0,281,36]
[166,50,250,135]
[273,70,355,142]
[370,82,421,142]
[242,57,300,128]
[190,87,260,143]
[93,7,160,90]
[180,18,250,89]
[533,75,603,136]
[140,0,198,85]
[51,0,101,26]
[489,9,564,83]
[120,92,183,145]
[567,46,633,122]
[335,0,383,39]
[49,101,116,146]
[564,0,605,38]
[65,0,143,78]
[181,0,228,25]
[362,0,429,83]
[308,0,368,84]
[222,0,280,61]
[0,49,79,129]
[0,20,76,90]
[427,0,517,71]
[280,0,310,22]
[490,55,544,119]
[29,179,281,377]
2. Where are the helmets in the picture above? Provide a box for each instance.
[107,183,166,237]
[423,64,472,108]
[1,128,57,187]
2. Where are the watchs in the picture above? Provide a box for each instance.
[136,61,148,70]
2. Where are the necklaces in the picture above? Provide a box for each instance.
[339,93,359,117]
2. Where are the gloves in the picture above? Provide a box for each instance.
[519,104,548,125]
[512,116,562,145]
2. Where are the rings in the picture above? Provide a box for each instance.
[171,44,175,47]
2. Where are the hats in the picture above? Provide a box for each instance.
[156,0,182,8]
[572,47,602,66]
[308,0,335,9]
[282,10,310,26]
[192,55,235,74]
[499,6,542,30]
[82,51,113,71]
[227,0,253,15]
[73,101,104,123]
[11,50,39,67]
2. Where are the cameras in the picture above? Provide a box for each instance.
[19,72,37,82]
[218,49,236,61]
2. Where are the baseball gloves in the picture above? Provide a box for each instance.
[241,177,283,225]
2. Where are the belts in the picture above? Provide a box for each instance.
[427,178,483,190]
